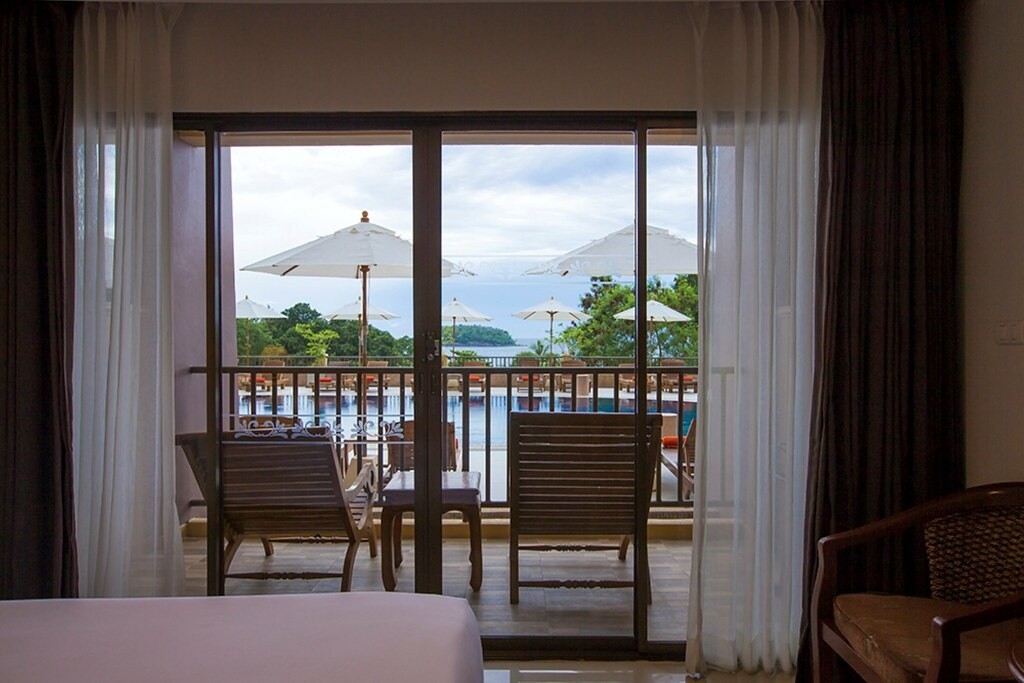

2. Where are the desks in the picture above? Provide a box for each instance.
[372,470,485,594]
[341,374,357,392]
[442,373,462,391]
[543,374,563,390]
[648,374,668,392]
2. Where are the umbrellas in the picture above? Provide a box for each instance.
[613,300,692,365]
[524,225,698,276]
[511,296,594,357]
[235,296,289,363]
[318,297,402,365]
[442,297,493,366]
[239,210,477,454]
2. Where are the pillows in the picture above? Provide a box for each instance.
[319,377,332,382]
[677,376,693,381]
[249,377,265,383]
[366,376,374,381]
[469,376,480,380]
[523,375,538,381]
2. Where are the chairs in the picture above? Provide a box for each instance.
[559,361,592,392]
[681,418,696,481]
[310,362,349,393]
[385,420,457,481]
[618,363,654,394]
[659,359,698,393]
[239,415,303,429]
[174,426,380,596]
[340,361,388,392]
[506,411,653,610]
[516,357,542,392]
[811,481,1024,683]
[456,362,486,392]
[241,360,285,392]
[409,356,449,393]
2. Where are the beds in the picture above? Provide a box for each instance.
[0,590,484,683]
